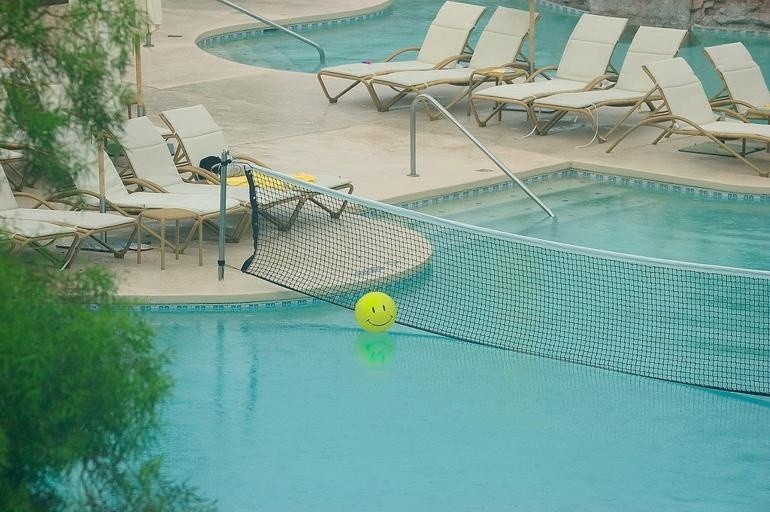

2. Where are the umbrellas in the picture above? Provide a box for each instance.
[65,0,161,249]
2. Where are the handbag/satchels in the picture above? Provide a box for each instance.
[198,156,222,180]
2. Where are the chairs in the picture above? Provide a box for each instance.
[469,12,642,136]
[48,124,248,254]
[318,1,490,112]
[0,84,119,191]
[369,6,556,122]
[2,166,136,268]
[530,26,688,144]
[138,208,203,269]
[665,41,770,139]
[1,217,78,270]
[158,105,353,230]
[605,57,770,178]
[104,116,303,242]
